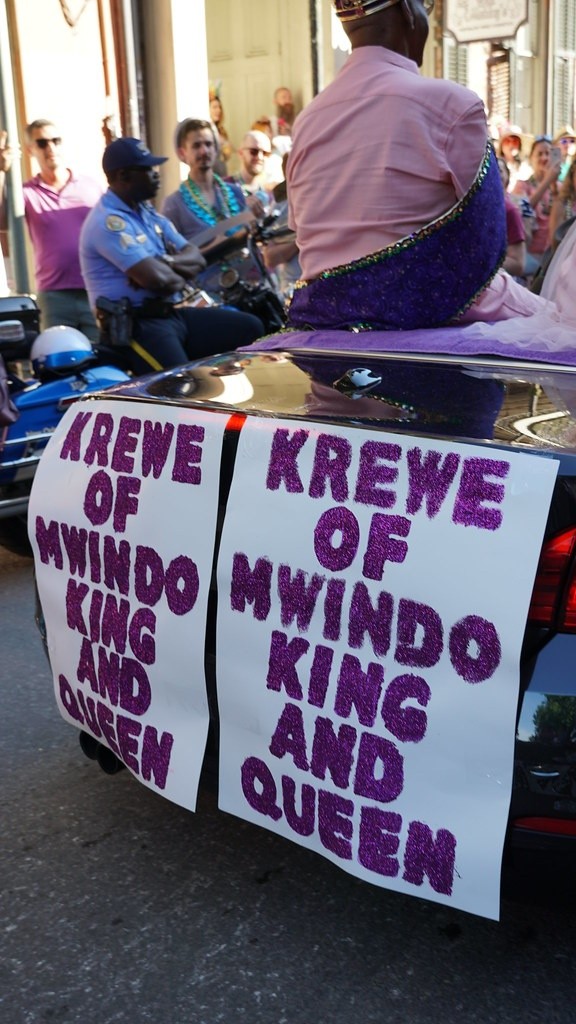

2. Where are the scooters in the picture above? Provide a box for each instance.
[0,207,289,558]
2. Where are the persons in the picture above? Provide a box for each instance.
[0,112,118,344]
[158,116,288,332]
[491,123,576,295]
[207,80,303,301]
[279,0,555,331]
[75,137,264,379]
[284,353,507,440]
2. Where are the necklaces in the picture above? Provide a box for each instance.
[530,176,552,215]
[566,199,572,220]
[178,172,243,236]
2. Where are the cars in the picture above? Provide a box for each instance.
[32,320,576,870]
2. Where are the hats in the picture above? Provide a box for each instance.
[103,137,169,172]
[497,125,534,158]
[553,127,576,144]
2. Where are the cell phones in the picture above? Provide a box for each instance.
[549,147,561,162]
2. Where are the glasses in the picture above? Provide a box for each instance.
[32,138,62,148]
[560,140,575,144]
[423,1,434,15]
[243,148,270,158]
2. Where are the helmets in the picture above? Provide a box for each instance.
[32,325,98,379]
